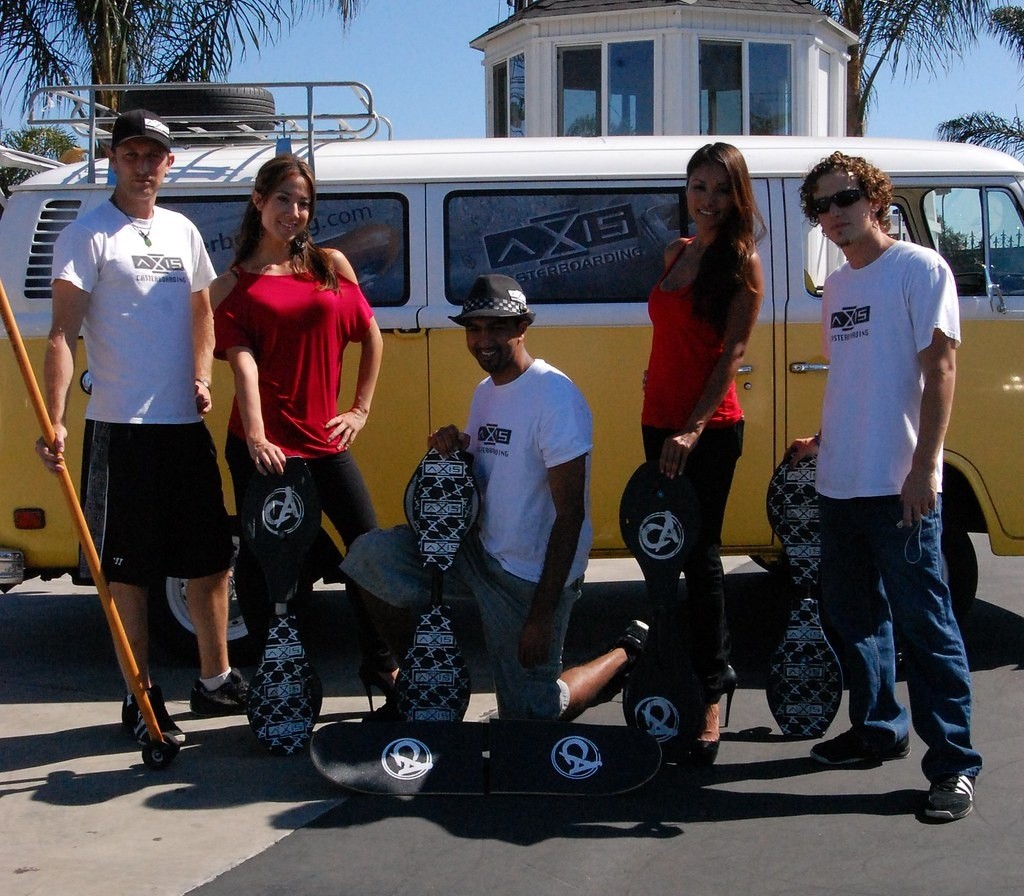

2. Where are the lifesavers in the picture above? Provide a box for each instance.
[110,82,276,133]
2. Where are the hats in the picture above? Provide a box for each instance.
[447,274,536,326]
[112,110,170,152]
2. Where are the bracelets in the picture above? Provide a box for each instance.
[814,434,820,447]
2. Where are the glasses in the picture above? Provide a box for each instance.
[807,188,864,213]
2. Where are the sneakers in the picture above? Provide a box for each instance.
[122,684,186,745]
[615,620,649,673]
[925,774,976,822]
[189,667,249,719]
[809,730,911,770]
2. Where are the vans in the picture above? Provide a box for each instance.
[0,78,1024,672]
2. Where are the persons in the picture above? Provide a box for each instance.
[36,109,253,747]
[641,142,767,755]
[340,274,650,722]
[213,154,405,712]
[782,153,982,819]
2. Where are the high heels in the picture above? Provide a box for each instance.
[358,658,394,712]
[720,665,737,727]
[697,735,720,775]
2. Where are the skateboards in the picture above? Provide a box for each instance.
[303,716,667,798]
[389,446,483,720]
[240,454,324,757]
[764,437,846,740]
[617,459,705,760]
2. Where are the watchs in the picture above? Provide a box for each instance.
[195,378,211,392]
